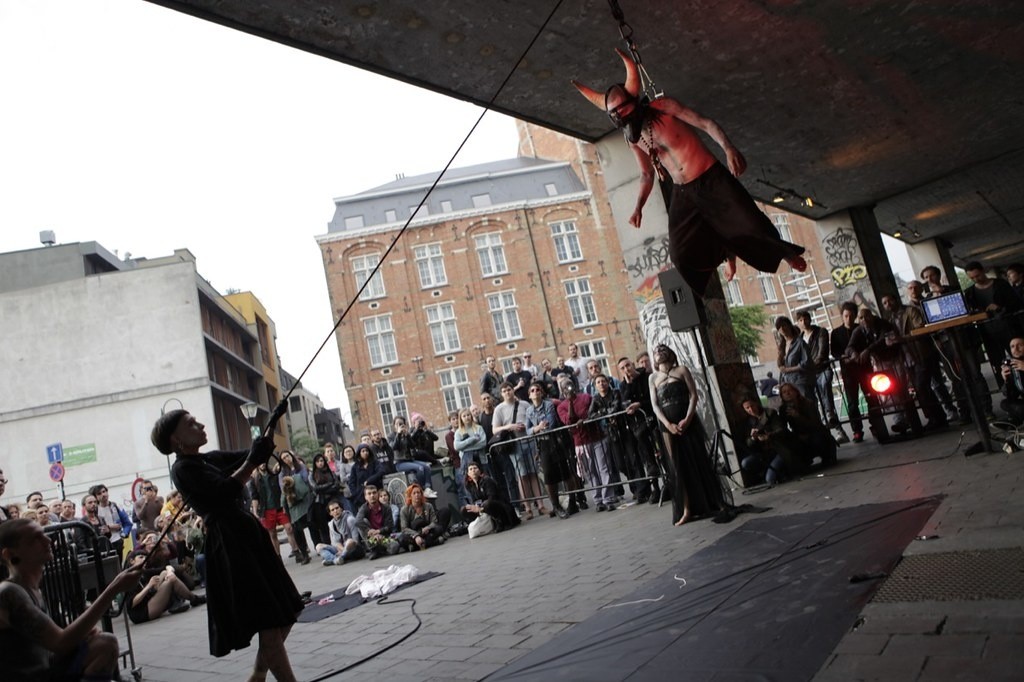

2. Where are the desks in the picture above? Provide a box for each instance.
[913,310,1019,460]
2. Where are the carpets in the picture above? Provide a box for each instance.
[488,490,943,682]
[293,569,446,619]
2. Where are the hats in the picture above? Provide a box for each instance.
[357,444,369,448]
[411,411,423,421]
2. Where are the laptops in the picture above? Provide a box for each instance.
[920,289,979,323]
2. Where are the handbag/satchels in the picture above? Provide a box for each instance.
[468,513,494,541]
[487,429,517,455]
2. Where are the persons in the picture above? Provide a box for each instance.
[442,342,734,534]
[735,256,1024,493]
[0,397,443,682]
[599,82,809,296]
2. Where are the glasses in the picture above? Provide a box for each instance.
[524,356,531,358]
[373,433,380,436]
[530,388,540,392]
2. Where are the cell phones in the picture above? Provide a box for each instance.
[147,487,151,491]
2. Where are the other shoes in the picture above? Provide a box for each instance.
[368,545,387,561]
[422,488,437,498]
[616,490,671,504]
[287,548,311,565]
[322,557,344,565]
[825,411,849,444]
[568,495,587,515]
[890,410,996,432]
[408,535,444,551]
[595,502,616,511]
[187,576,206,591]
[989,417,1024,431]
[168,595,207,614]
[514,501,548,520]
[549,502,568,518]
[852,425,878,442]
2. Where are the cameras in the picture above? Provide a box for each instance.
[419,420,425,428]
[566,385,574,395]
[1002,359,1011,366]
